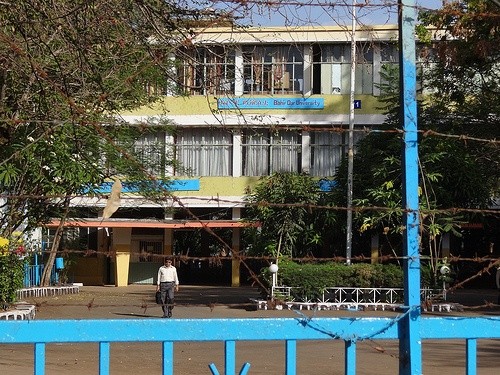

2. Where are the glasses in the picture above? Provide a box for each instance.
[165,261,171,263]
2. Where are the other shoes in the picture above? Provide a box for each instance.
[161,313,172,318]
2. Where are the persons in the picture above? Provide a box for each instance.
[157,257,179,318]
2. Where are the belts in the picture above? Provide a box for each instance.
[161,282,175,283]
